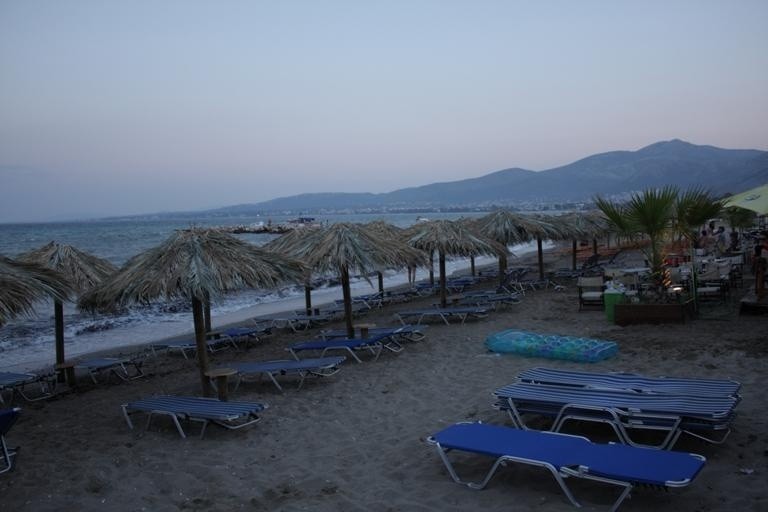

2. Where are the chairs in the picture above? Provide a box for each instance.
[119,392,277,445]
[491,365,743,459]
[423,420,710,510]
[3,351,145,500]
[150,267,598,391]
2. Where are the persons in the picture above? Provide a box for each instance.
[700,221,768,291]
